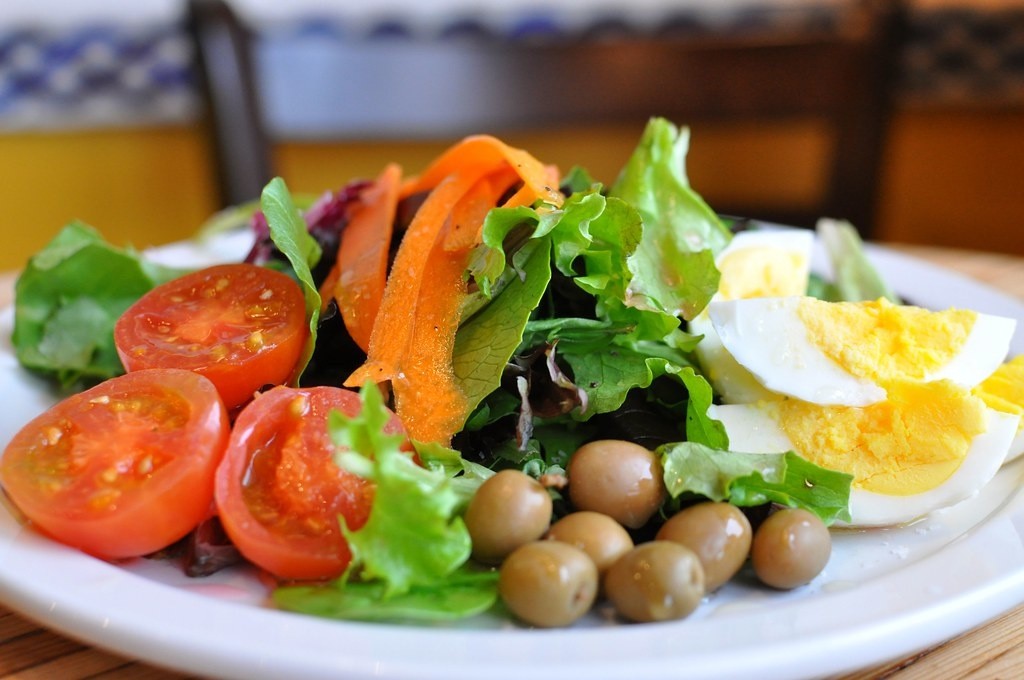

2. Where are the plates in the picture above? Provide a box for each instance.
[1,228,1024,679]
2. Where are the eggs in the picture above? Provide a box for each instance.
[682,227,1024,528]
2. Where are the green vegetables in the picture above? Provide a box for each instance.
[271,117,856,621]
[8,176,330,399]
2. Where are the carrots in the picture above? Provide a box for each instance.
[444,177,494,250]
[338,160,399,351]
[346,163,498,382]
[477,165,561,243]
[316,262,338,319]
[392,163,519,445]
[397,131,565,206]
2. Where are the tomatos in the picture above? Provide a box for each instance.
[114,261,308,405]
[214,385,427,583]
[1,368,235,556]
